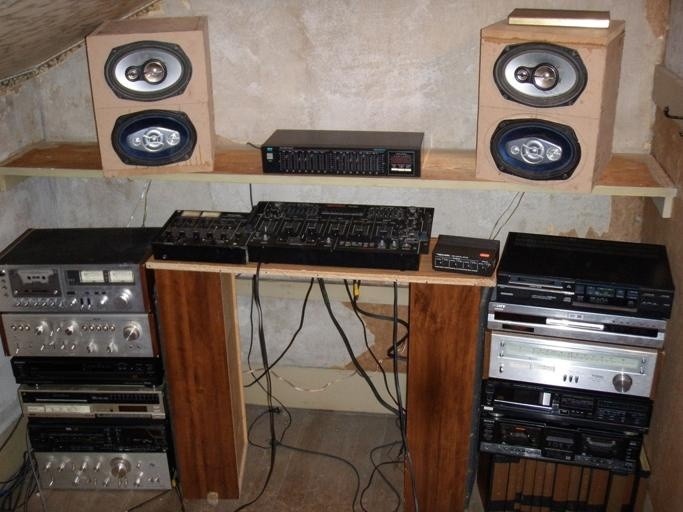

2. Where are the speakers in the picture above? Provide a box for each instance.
[83,15,217,176]
[473,18,627,196]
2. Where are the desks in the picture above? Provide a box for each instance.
[142,236,498,511]
